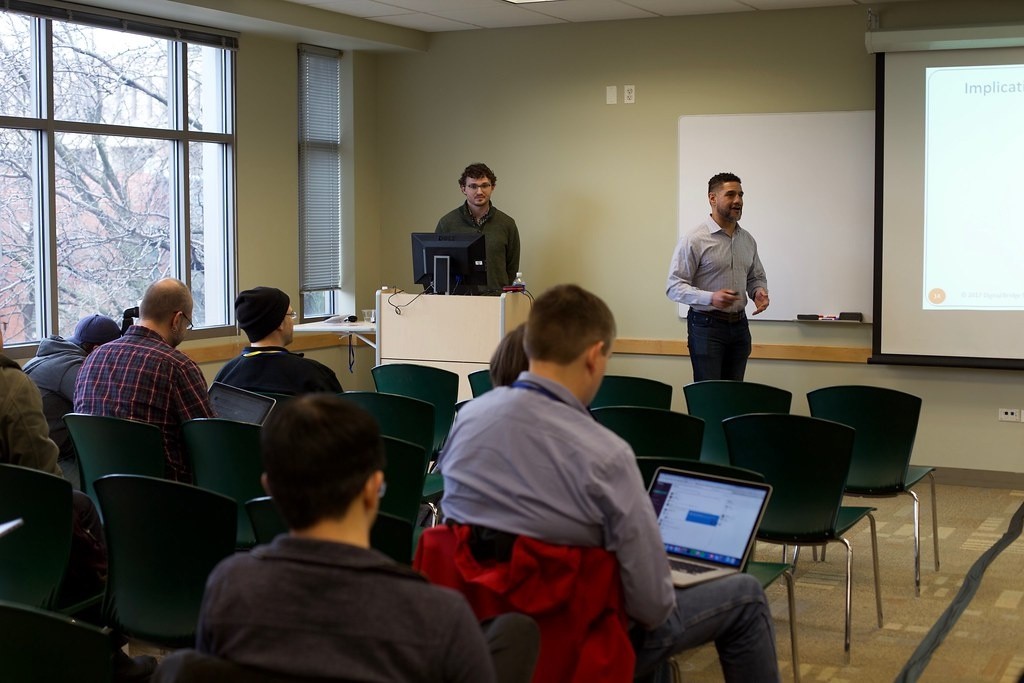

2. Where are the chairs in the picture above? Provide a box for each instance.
[0,362,940,683]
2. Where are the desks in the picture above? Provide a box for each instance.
[293,322,376,350]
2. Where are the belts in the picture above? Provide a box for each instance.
[691,306,746,321]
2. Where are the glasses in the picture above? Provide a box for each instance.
[463,183,492,188]
[173,311,193,330]
[286,311,297,319]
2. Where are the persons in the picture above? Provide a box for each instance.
[72,278,221,474]
[489,320,530,388]
[195,391,538,683]
[208,286,346,397]
[0,329,106,546]
[435,162,520,297]
[666,172,769,382]
[18,314,121,492]
[437,284,781,683]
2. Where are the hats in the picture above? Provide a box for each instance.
[67,314,121,344]
[235,287,290,343]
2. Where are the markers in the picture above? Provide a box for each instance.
[819,316,838,320]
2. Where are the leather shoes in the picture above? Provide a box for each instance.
[111,647,157,683]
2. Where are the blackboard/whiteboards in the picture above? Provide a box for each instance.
[677,110,876,324]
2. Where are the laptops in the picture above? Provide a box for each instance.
[208,381,276,426]
[645,466,773,587]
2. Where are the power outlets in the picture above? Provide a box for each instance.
[624,84,635,103]
[999,408,1020,422]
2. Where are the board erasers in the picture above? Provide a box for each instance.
[796,315,819,320]
[838,312,863,323]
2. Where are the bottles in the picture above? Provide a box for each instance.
[513,272,526,295]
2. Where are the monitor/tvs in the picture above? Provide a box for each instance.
[410,232,488,297]
